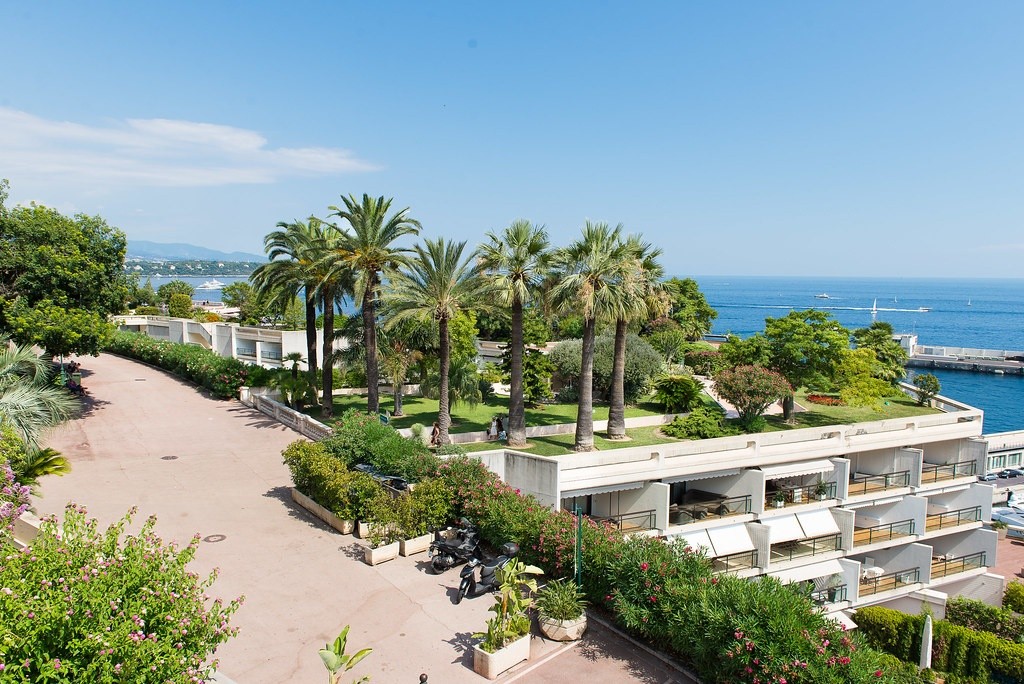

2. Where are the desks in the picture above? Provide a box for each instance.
[781,484,803,503]
[862,564,884,581]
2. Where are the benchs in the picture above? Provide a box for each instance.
[670,489,729,525]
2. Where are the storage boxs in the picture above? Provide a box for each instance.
[504,542,520,558]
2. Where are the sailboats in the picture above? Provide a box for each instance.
[967,298,974,307]
[869,299,877,315]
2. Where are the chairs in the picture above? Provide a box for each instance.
[860,557,877,587]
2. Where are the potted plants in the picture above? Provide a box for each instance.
[992,519,1009,539]
[471,556,545,681]
[279,438,453,566]
[815,480,828,500]
[532,580,593,641]
[772,491,785,507]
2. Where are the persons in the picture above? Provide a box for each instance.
[489,416,503,440]
[1001,488,1014,508]
[430,422,439,445]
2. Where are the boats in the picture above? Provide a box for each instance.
[815,294,829,300]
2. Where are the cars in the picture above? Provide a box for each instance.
[980,473,1000,481]
[998,468,1024,480]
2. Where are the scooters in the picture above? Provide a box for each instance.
[430,516,486,575]
[462,539,521,604]
